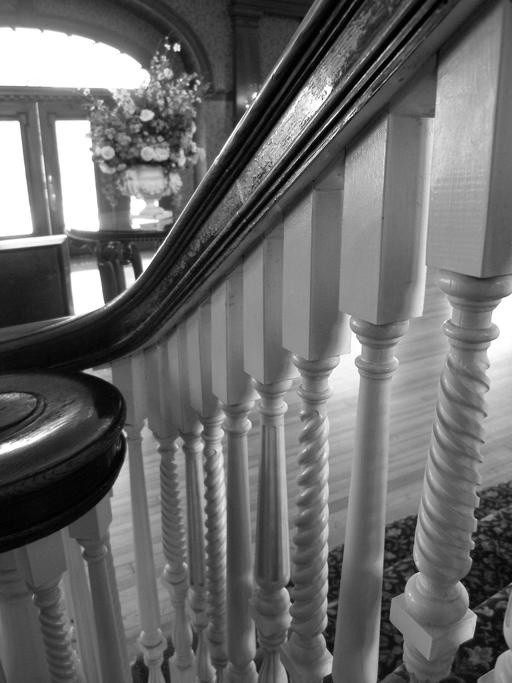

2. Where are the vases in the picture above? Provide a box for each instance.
[114,164,182,231]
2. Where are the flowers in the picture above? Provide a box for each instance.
[78,35,207,174]
